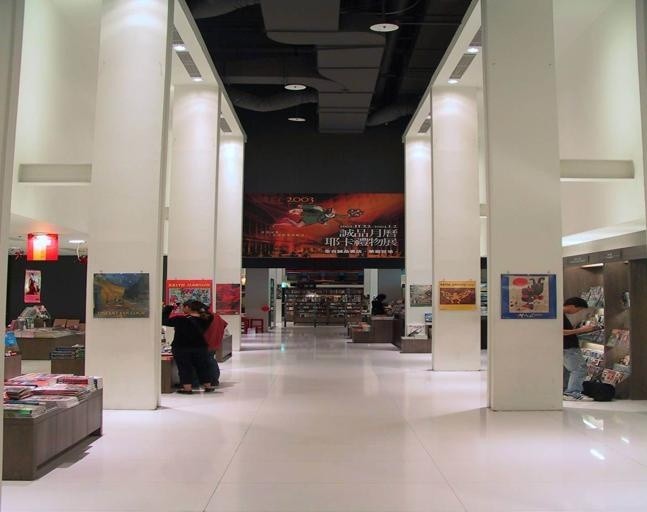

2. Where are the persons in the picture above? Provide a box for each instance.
[161,298,220,395]
[26,272,37,295]
[562,298,597,401]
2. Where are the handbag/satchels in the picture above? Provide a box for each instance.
[581,381,615,401]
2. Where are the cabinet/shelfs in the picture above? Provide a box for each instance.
[214,329,233,363]
[283,286,405,349]
[240,316,263,334]
[0,317,103,481]
[161,342,180,393]
[401,313,432,353]
[563,245,647,400]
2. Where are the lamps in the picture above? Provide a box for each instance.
[367,0,400,34]
[287,105,307,123]
[284,83,307,91]
[27,233,58,262]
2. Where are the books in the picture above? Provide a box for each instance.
[573,287,631,384]
[270,281,431,338]
[2,303,174,417]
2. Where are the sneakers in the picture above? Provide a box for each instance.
[177,390,192,394]
[205,388,215,392]
[563,392,594,401]
